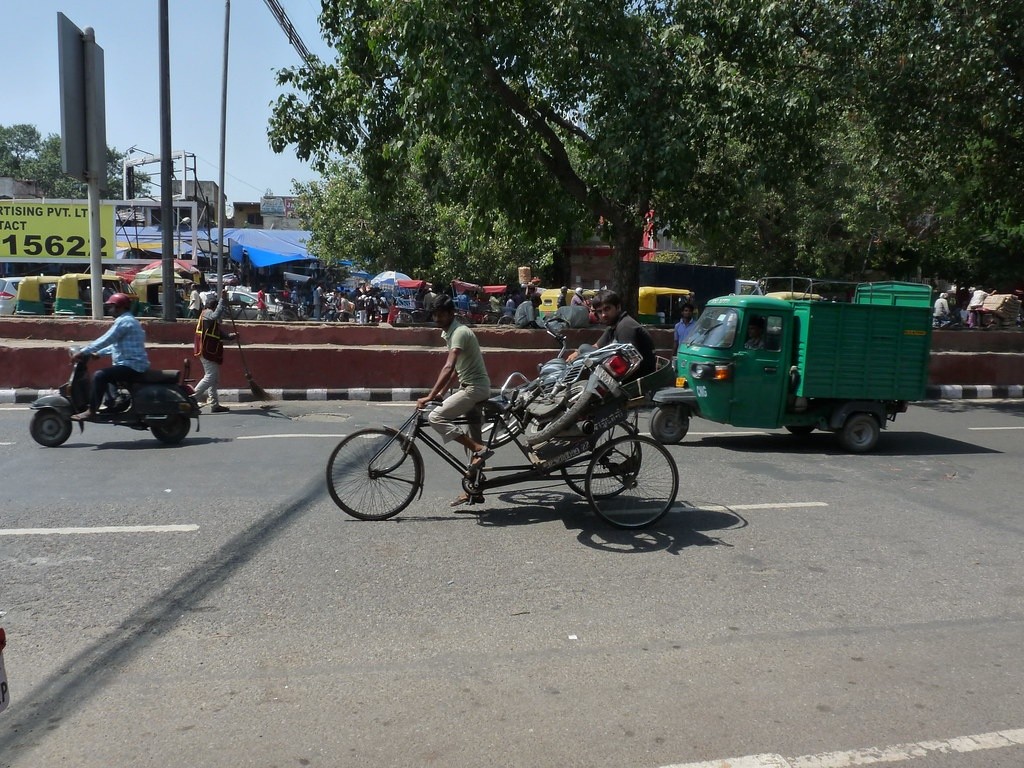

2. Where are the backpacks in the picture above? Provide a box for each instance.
[366,297,374,310]
[357,296,366,310]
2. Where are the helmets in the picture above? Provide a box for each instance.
[105,293,131,311]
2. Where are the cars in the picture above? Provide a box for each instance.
[0,278,21,315]
[224,291,284,322]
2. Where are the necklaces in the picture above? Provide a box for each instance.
[256,286,269,320]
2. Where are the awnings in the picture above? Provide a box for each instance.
[228,229,353,268]
[130,225,240,259]
[115,226,144,259]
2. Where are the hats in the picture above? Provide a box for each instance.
[205,299,218,308]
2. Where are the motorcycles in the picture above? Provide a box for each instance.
[765,292,827,302]
[469,318,644,449]
[15,276,62,315]
[130,279,205,319]
[28,341,201,449]
[638,287,698,327]
[538,288,593,326]
[55,273,140,316]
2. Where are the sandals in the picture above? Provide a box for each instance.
[70,411,96,420]
[450,493,470,506]
[467,446,495,472]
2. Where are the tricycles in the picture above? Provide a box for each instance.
[325,315,680,530]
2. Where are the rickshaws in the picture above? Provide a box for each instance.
[269,285,356,323]
[367,272,538,327]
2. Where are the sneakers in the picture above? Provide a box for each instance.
[211,406,230,414]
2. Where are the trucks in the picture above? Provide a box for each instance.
[649,277,934,455]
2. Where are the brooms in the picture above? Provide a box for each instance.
[220,290,277,401]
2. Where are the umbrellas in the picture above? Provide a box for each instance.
[141,258,201,277]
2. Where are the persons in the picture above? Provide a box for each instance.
[489,293,503,317]
[556,286,568,308]
[271,280,485,326]
[670,303,698,359]
[548,290,655,441]
[70,294,150,421]
[187,284,201,319]
[515,295,547,329]
[506,294,516,324]
[933,292,951,325]
[190,290,229,411]
[966,285,997,330]
[744,321,766,350]
[570,287,595,312]
[46,284,56,298]
[418,294,495,506]
